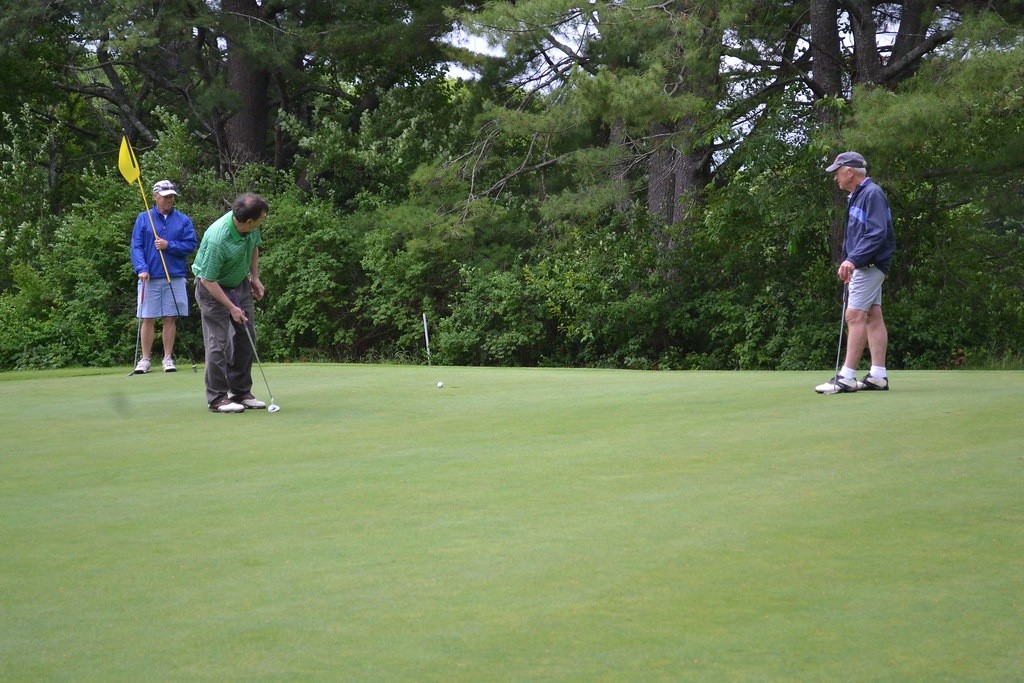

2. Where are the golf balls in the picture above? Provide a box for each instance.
[436,380,444,390]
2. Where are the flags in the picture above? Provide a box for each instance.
[119,135,140,184]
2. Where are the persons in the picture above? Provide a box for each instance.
[192,193,269,413]
[130,179,198,372]
[815,152,896,394]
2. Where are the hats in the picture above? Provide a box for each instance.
[825,152,866,172]
[153,180,177,197]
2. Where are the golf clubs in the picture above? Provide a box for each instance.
[239,308,282,412]
[823,271,849,394]
[128,278,147,377]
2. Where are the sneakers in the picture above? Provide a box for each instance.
[240,393,266,409]
[135,359,152,373]
[857,371,889,390]
[161,355,176,371]
[815,373,859,393]
[209,399,245,413]
